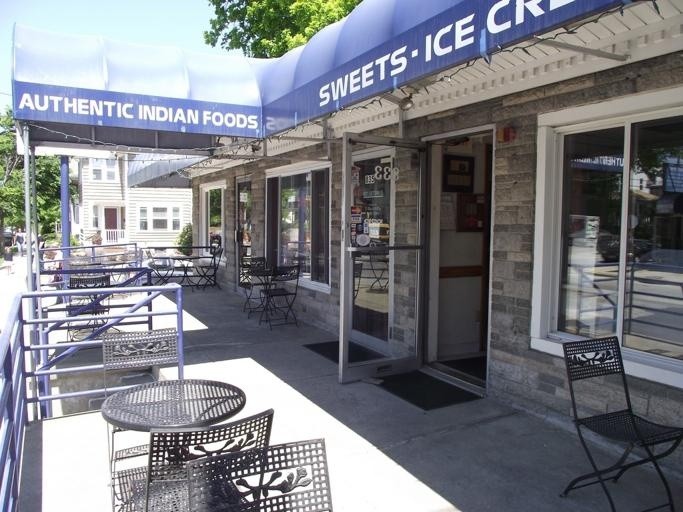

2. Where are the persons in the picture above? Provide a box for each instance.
[13,228,25,257]
[38,233,45,271]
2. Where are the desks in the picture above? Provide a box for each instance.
[351,246,388,288]
[101,378,246,507]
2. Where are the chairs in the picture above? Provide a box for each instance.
[185,438,333,511]
[354,263,363,297]
[114,408,274,512]
[53,247,303,342]
[560,339,683,512]
[369,242,390,290]
